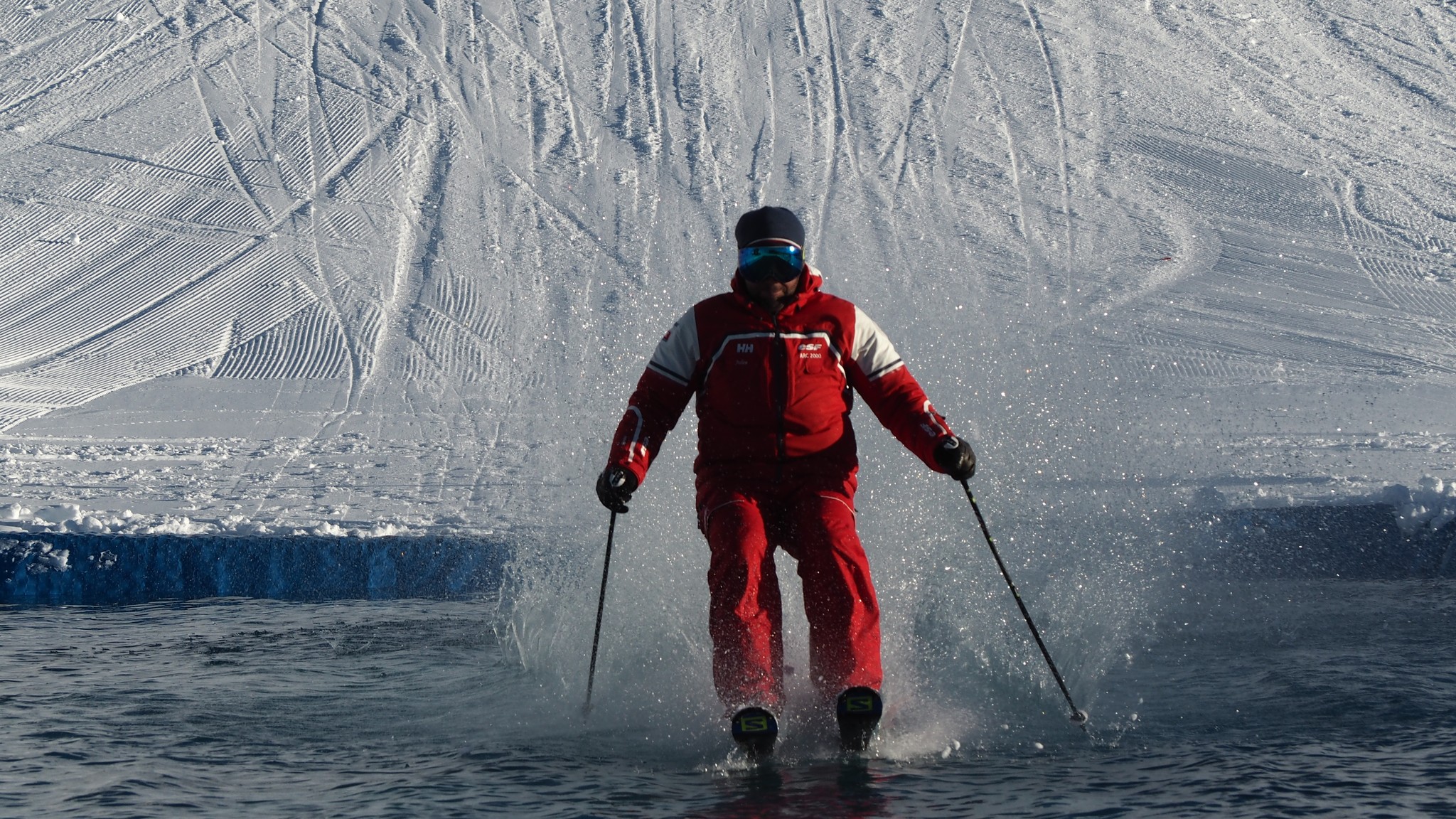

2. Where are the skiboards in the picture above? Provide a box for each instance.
[731,682,884,761]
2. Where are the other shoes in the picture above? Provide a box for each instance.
[836,685,882,751]
[731,707,779,756]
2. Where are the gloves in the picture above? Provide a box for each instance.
[596,462,640,514]
[932,434,976,481]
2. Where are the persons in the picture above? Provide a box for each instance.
[598,205,976,752]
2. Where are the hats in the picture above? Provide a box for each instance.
[734,207,804,287]
